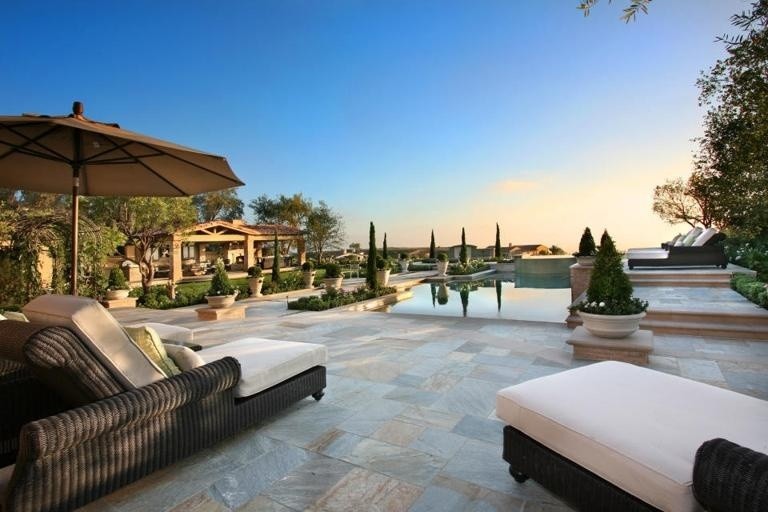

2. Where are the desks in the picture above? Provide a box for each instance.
[159,339,201,353]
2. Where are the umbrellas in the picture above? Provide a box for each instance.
[1,100,248,298]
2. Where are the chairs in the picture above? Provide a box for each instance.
[496,357,767,512]
[0,318,327,510]
[627,232,728,269]
[122,324,195,345]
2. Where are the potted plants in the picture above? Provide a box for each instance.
[203,254,455,307]
[567,225,649,338]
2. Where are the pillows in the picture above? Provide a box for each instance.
[673,228,717,247]
[22,294,206,391]
